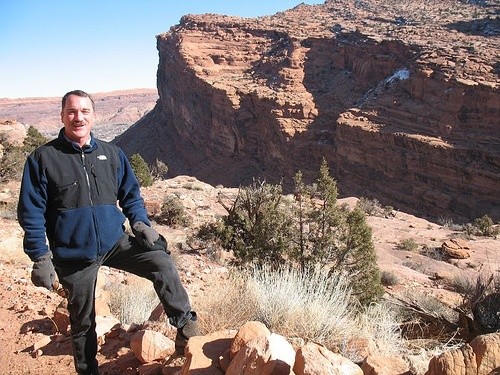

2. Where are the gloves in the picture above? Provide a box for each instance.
[133,221,160,245]
[31,251,55,291]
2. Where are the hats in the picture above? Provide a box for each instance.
[134,230,172,255]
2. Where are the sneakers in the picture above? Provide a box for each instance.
[174,321,205,355]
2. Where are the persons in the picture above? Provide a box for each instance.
[16,90,199,374]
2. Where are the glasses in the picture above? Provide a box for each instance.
[52,280,68,298]
